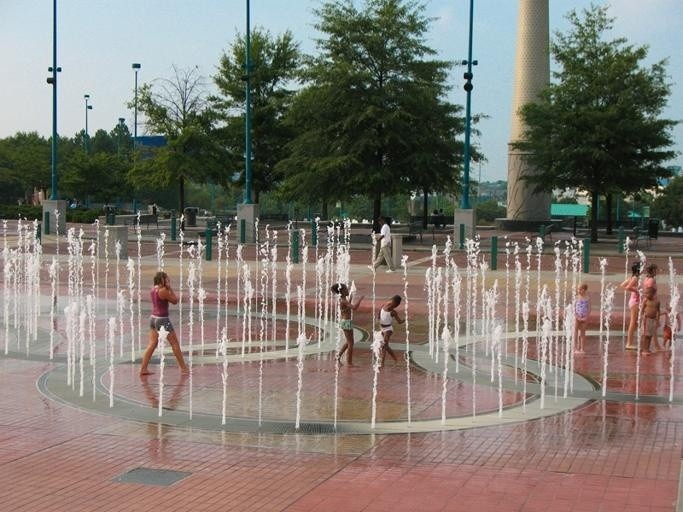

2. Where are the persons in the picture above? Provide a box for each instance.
[619,263,681,356]
[569,356,594,373]
[70,198,158,216]
[138,271,193,377]
[366,218,396,273]
[329,284,364,369]
[430,208,446,229]
[376,294,413,369]
[624,350,673,428]
[142,375,189,474]
[570,283,593,354]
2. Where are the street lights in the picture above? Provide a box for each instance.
[84,95,92,208]
[42,66,67,236]
[116,118,125,209]
[235,74,261,244]
[131,63,140,214]
[452,59,478,250]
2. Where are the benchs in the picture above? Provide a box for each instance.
[207,212,234,235]
[530,224,555,243]
[410,217,456,228]
[407,221,435,242]
[123,213,159,233]
[633,225,651,249]
